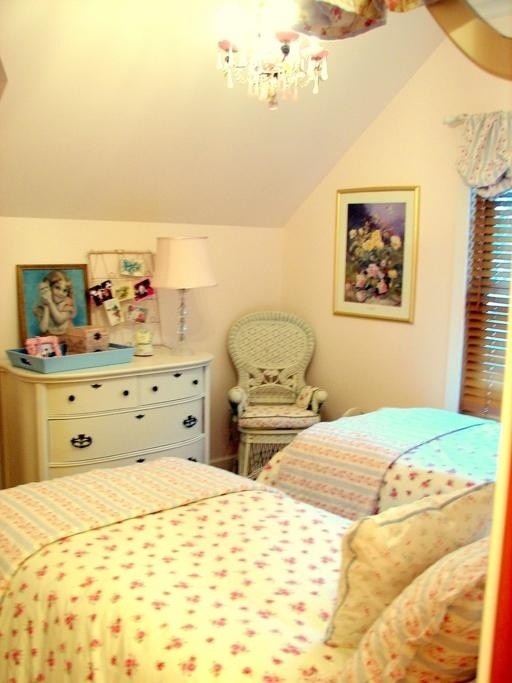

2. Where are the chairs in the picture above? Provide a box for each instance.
[226,309,328,481]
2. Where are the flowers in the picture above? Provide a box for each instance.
[345,204,402,307]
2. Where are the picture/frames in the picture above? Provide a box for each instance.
[16,264,92,347]
[333,184,421,325]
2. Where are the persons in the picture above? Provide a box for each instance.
[93,285,112,305]
[33,271,76,337]
[136,285,147,300]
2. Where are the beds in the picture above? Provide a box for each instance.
[253,405,501,521]
[0,452,366,682]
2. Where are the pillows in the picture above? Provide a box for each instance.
[326,533,491,683]
[322,481,495,647]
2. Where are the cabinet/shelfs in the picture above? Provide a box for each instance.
[0,345,214,487]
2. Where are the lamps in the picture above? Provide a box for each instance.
[147,234,218,360]
[213,1,330,111]
